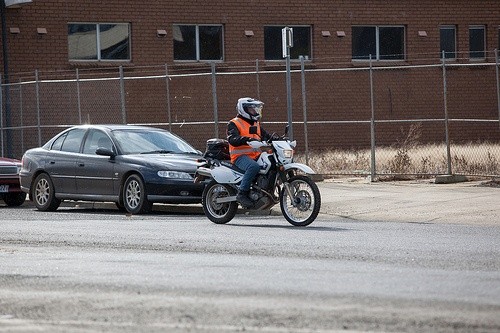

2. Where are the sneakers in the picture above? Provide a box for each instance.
[236,194,254,206]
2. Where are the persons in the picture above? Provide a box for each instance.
[225,97,292,210]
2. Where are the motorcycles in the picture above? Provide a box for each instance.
[194,125,321,226]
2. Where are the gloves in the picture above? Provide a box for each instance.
[249,137,258,142]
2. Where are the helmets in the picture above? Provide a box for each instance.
[236,97,264,121]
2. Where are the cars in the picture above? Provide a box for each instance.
[19,124,211,214]
[0,156,26,206]
